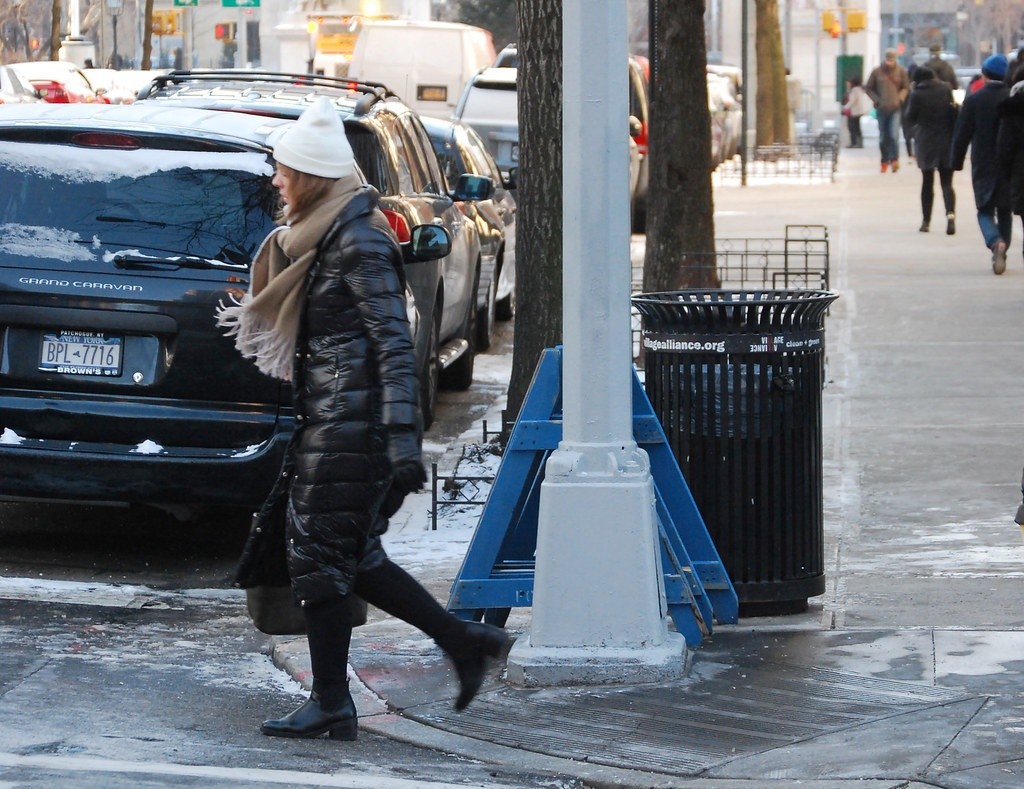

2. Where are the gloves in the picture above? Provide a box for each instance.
[394,461,426,493]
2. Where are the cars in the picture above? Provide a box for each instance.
[0,58,177,108]
[949,67,981,110]
[454,40,742,232]
[909,50,963,71]
[419,111,516,353]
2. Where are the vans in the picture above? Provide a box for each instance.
[346,21,495,120]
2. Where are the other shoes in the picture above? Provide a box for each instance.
[992,238,1006,273]
[920,220,929,231]
[946,212,955,235]
[881,162,887,172]
[892,160,898,171]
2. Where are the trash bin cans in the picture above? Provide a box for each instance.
[631,287,841,619]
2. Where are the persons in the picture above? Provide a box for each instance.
[217,98,513,744]
[837,43,1024,276]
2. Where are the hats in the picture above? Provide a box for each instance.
[884,48,896,60]
[979,53,1007,80]
[928,42,942,52]
[271,96,354,179]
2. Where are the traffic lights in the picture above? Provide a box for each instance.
[214,22,233,40]
[105,0,124,71]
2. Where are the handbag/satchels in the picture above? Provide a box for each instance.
[247,511,367,635]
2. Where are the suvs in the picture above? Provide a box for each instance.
[130,68,500,433]
[1,104,452,513]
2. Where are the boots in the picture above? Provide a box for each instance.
[848,135,855,147]
[259,593,358,740]
[854,134,863,148]
[350,557,506,713]
